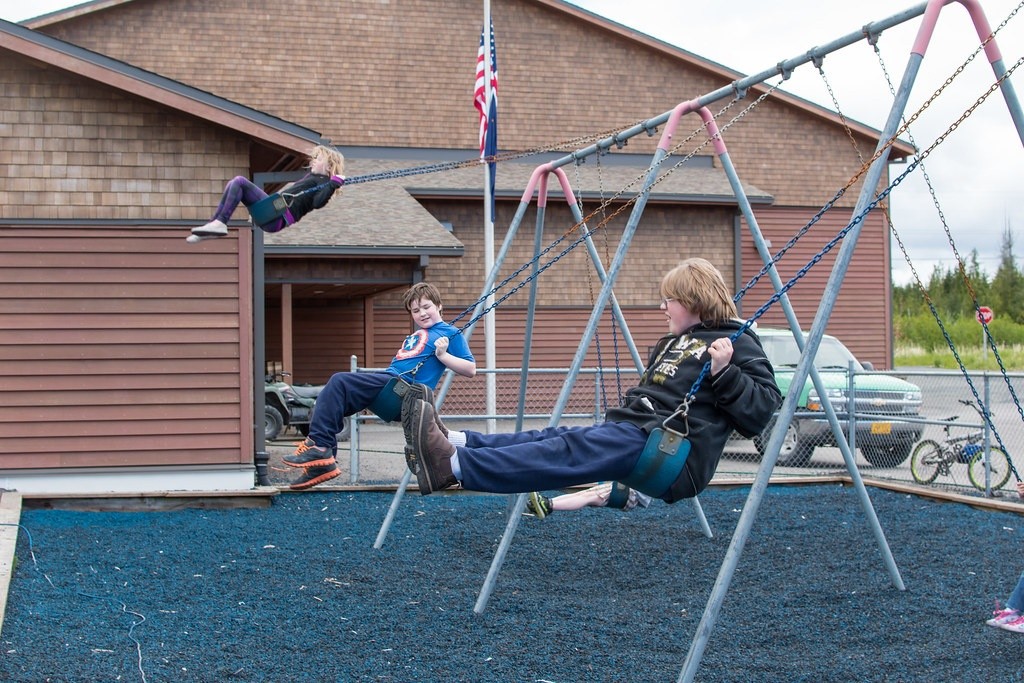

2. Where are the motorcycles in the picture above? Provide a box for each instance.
[263,371,366,444]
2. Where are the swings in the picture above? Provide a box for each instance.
[563,143,636,510]
[613,0,1024,500]
[372,74,788,424]
[247,123,635,229]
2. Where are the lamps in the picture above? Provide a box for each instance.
[753,240,772,249]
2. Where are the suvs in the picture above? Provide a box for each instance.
[750,328,927,468]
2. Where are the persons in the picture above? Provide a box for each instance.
[400,258,782,495]
[282,282,476,489]
[185,145,346,243]
[527,483,652,517]
[987,483,1024,633]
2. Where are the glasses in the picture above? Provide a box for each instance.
[661,298,678,310]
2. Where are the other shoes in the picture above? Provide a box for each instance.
[191,221,231,236]
[402,383,449,474]
[186,234,221,243]
[412,400,462,495]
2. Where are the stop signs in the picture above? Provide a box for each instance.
[976,306,994,325]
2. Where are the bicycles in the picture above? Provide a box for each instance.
[911,398,1014,493]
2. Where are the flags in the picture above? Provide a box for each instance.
[474,15,497,224]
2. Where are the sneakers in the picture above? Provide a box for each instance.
[290,462,342,490]
[526,498,537,515]
[282,437,335,468]
[530,492,552,519]
